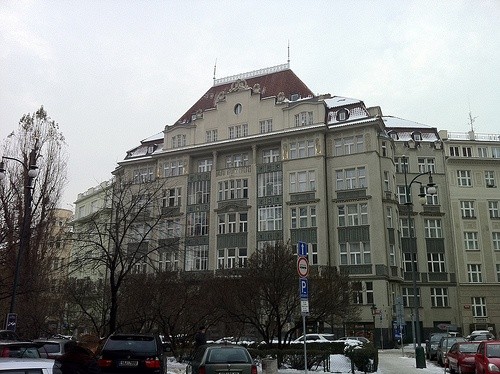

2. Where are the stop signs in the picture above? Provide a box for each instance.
[297,257,309,278]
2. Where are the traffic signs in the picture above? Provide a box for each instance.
[298,279,309,313]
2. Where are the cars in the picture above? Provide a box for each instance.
[466,331,496,341]
[339,336,371,344]
[437,337,468,364]
[186,344,257,374]
[475,340,500,374]
[447,342,481,374]
[449,332,463,337]
[0,329,77,374]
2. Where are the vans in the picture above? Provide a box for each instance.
[293,334,338,343]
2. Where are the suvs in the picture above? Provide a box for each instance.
[94,331,167,374]
[425,332,451,361]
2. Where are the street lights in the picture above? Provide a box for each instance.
[401,154,436,369]
[0,156,39,313]
